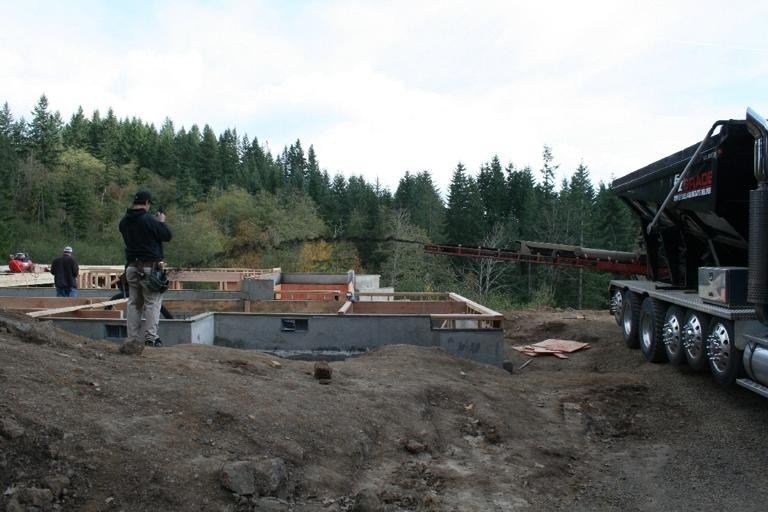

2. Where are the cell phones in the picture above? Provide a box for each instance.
[158,205,163,213]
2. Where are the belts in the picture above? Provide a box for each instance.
[129,260,153,267]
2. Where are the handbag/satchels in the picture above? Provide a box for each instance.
[146,269,170,293]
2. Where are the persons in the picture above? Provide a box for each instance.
[119,191,173,346]
[50,247,79,297]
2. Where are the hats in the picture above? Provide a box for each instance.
[64,246,72,252]
[135,191,154,203]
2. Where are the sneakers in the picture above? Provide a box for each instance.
[146,338,165,347]
